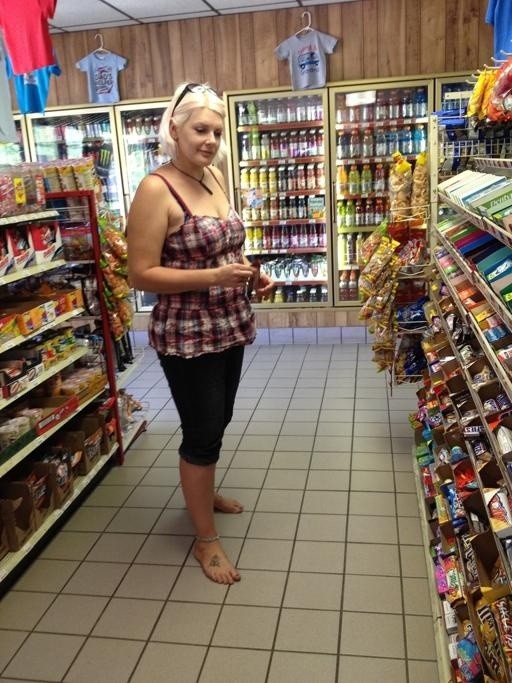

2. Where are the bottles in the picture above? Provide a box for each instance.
[337,163,390,226]
[125,115,161,134]
[337,89,427,158]
[337,232,365,300]
[237,94,327,302]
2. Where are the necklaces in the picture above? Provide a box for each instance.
[169,156,216,196]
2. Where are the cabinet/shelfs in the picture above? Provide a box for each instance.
[390,113,512,683]
[0,184,147,600]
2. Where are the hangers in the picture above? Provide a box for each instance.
[93,34,111,54]
[294,11,314,37]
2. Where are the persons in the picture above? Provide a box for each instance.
[124,80,275,586]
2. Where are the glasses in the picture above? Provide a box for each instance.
[169,82,221,118]
